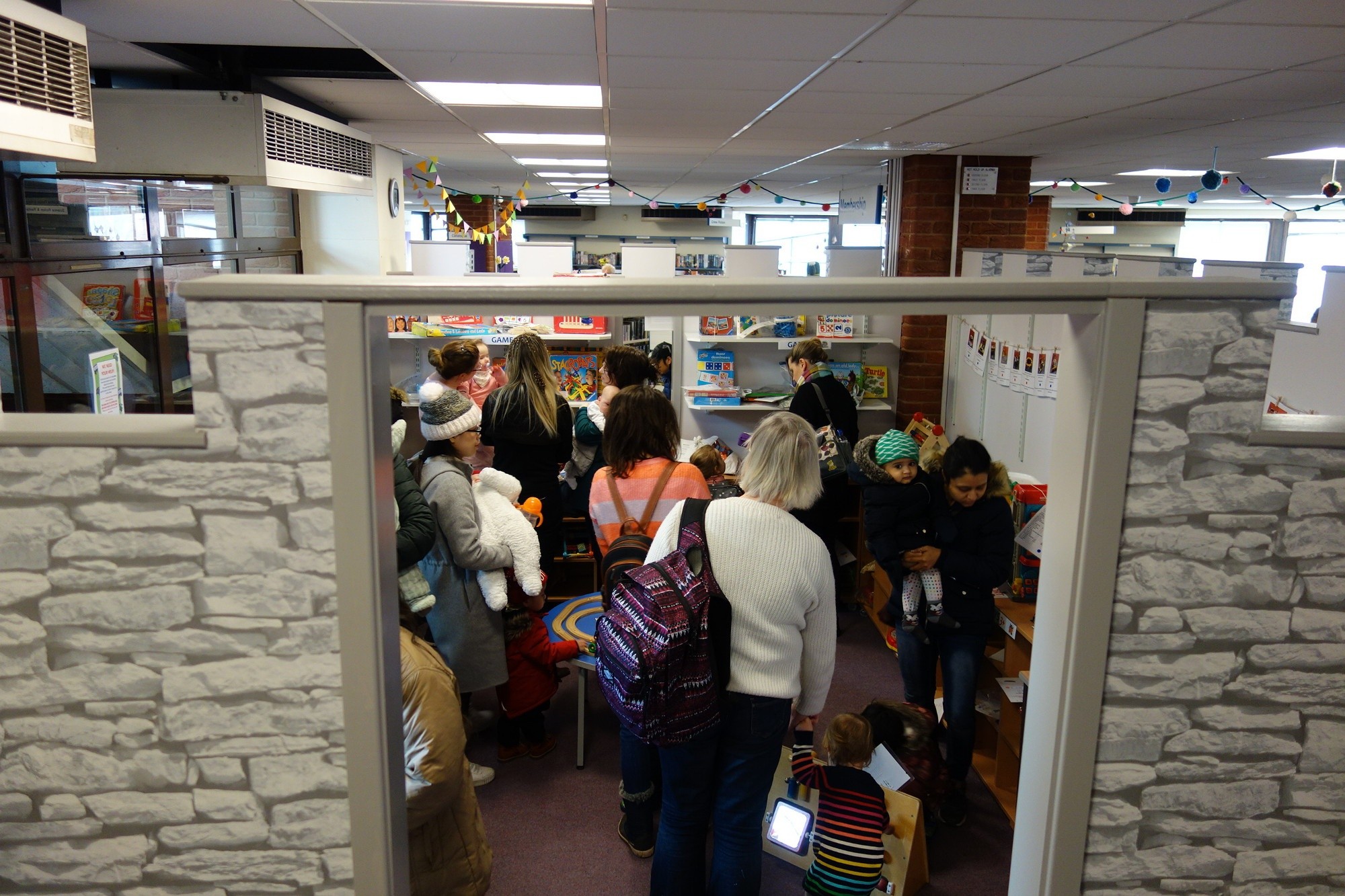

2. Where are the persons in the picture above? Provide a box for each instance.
[588,384,714,858]
[554,370,566,392]
[790,714,896,896]
[690,445,744,499]
[498,565,587,762]
[858,697,954,816]
[786,338,859,578]
[871,435,1017,825]
[468,466,543,612]
[415,332,672,559]
[387,316,395,332]
[391,397,438,656]
[404,381,509,789]
[642,410,838,896]
[855,430,943,635]
[581,369,596,396]
[394,316,407,332]
[399,625,493,896]
[406,315,418,332]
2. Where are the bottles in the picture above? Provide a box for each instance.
[516,497,543,529]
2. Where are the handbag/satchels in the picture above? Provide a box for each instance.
[811,383,852,481]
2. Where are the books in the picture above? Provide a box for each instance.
[575,251,621,266]
[622,316,651,355]
[676,253,724,277]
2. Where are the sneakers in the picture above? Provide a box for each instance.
[466,706,494,734]
[529,733,556,759]
[497,741,529,763]
[937,780,968,827]
[468,761,495,786]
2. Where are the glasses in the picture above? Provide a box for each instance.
[466,427,481,436]
[469,363,482,374]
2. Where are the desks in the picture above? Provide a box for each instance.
[542,592,603,768]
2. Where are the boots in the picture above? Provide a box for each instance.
[618,780,658,858]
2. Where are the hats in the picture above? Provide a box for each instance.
[503,564,547,603]
[875,429,920,466]
[418,378,482,441]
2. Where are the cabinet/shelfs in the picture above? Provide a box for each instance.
[688,335,894,411]
[389,331,613,409]
[546,516,597,600]
[841,488,1033,834]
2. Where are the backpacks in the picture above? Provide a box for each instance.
[594,498,732,745]
[600,460,680,613]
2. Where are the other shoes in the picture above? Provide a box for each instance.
[408,594,436,617]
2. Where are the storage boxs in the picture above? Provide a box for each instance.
[685,350,742,405]
[796,315,807,335]
[828,361,888,398]
[387,315,610,401]
[80,277,182,333]
[817,314,854,338]
